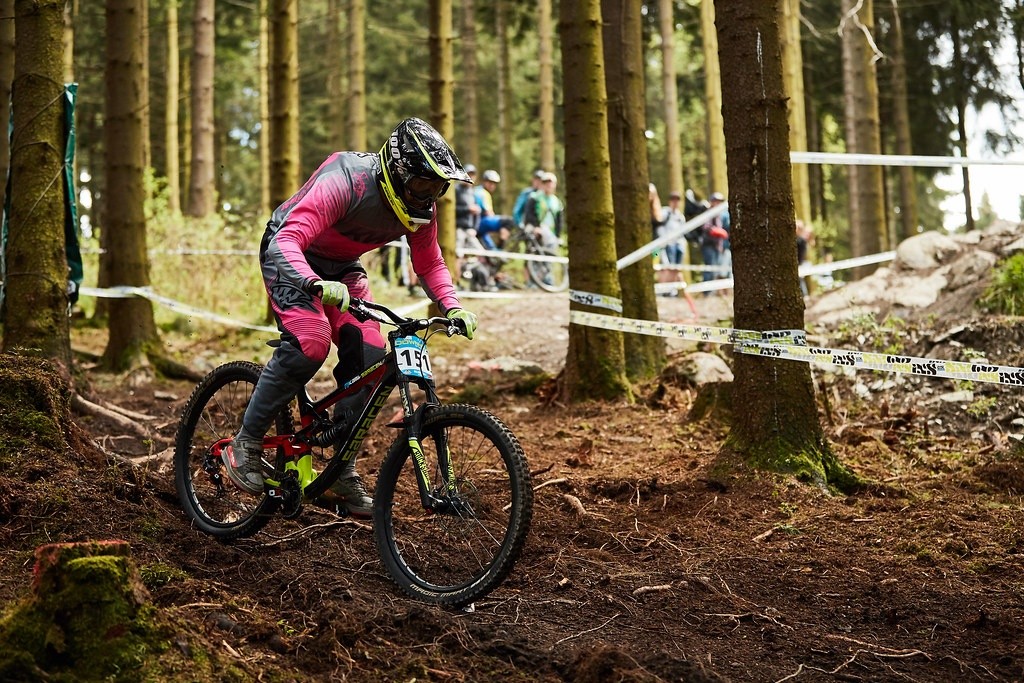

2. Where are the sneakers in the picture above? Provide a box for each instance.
[221,424,265,496]
[321,458,373,515]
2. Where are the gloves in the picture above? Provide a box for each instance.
[446,307,479,341]
[310,280,349,314]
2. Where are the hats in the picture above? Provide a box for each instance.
[535,169,545,178]
[464,163,477,176]
[541,172,558,183]
[668,191,681,200]
[710,192,725,201]
[483,169,501,183]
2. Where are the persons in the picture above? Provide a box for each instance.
[221,117,475,517]
[647,183,731,297]
[796,222,836,308]
[456,163,564,291]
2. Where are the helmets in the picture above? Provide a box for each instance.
[376,116,474,233]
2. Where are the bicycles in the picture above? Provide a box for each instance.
[380,220,570,296]
[172,288,534,609]
